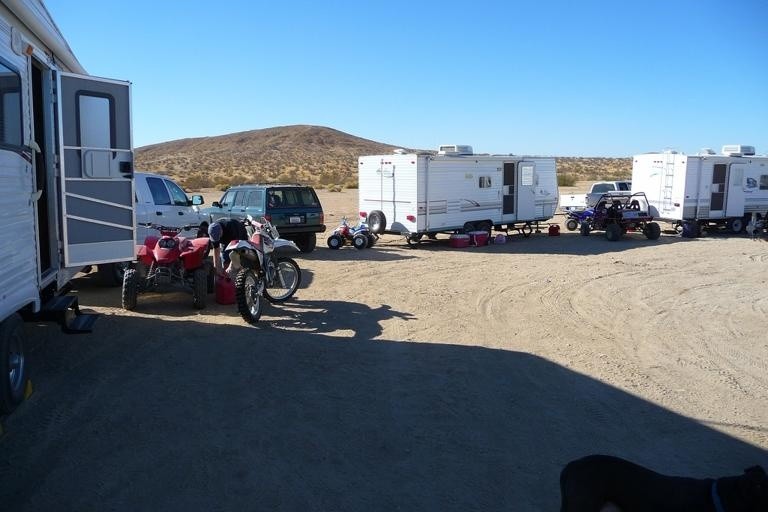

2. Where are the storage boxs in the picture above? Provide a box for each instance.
[451,235,470,248]
[469,231,488,246]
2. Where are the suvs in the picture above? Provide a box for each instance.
[119,217,215,310]
[93,170,217,286]
[563,206,597,231]
[326,215,379,251]
[196,179,327,255]
[589,190,663,243]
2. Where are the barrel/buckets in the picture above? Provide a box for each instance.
[216,274,235,306]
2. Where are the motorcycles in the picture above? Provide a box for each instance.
[225,213,304,325]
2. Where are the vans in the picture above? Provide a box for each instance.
[590,179,632,193]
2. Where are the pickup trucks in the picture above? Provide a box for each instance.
[585,191,631,207]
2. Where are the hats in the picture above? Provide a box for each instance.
[207,223,221,242]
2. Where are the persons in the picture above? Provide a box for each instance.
[208,217,247,274]
